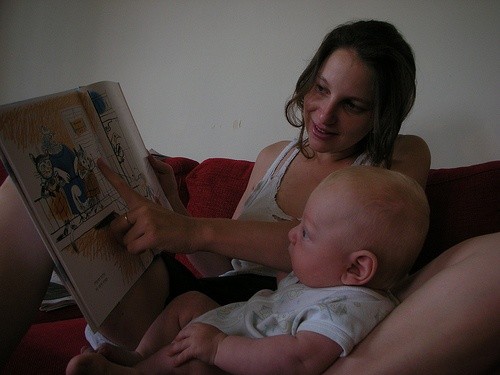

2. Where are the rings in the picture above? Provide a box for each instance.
[123,212,134,227]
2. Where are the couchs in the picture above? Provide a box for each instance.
[2,153,500,374]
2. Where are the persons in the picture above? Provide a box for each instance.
[63,166,431,375]
[0,19,500,375]
